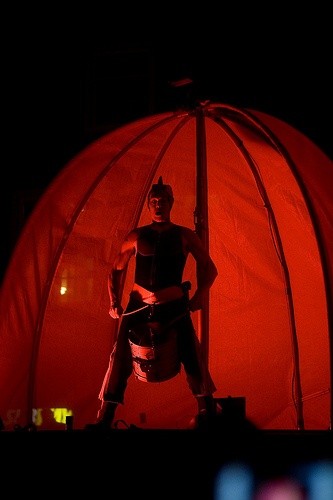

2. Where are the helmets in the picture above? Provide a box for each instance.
[147,175,173,203]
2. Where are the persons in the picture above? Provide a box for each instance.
[94,177,220,428]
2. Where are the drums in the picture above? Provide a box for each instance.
[127,321,182,384]
